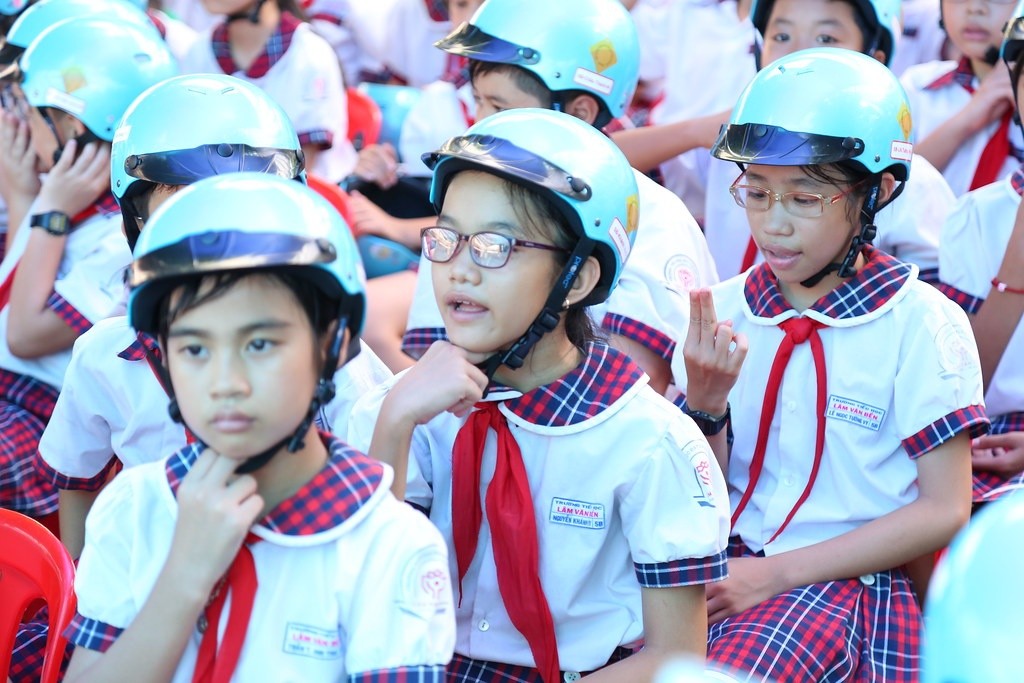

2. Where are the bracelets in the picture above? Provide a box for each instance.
[990,277,1024,294]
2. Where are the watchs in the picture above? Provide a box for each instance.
[30,208,73,236]
[686,400,731,436]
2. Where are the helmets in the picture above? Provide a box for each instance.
[128,171,368,401]
[0,0,182,142]
[110,72,308,211]
[998,0,1024,64]
[709,47,914,182]
[749,0,903,71]
[433,0,643,119]
[422,107,640,308]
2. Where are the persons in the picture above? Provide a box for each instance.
[57,172,460,683]
[0,0,1024,683]
[635,45,992,683]
[346,106,732,683]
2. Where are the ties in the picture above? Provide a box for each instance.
[0,205,100,312]
[192,530,264,683]
[731,315,830,542]
[451,401,561,683]
[968,108,1014,191]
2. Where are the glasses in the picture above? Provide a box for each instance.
[729,170,868,219]
[420,227,573,269]
